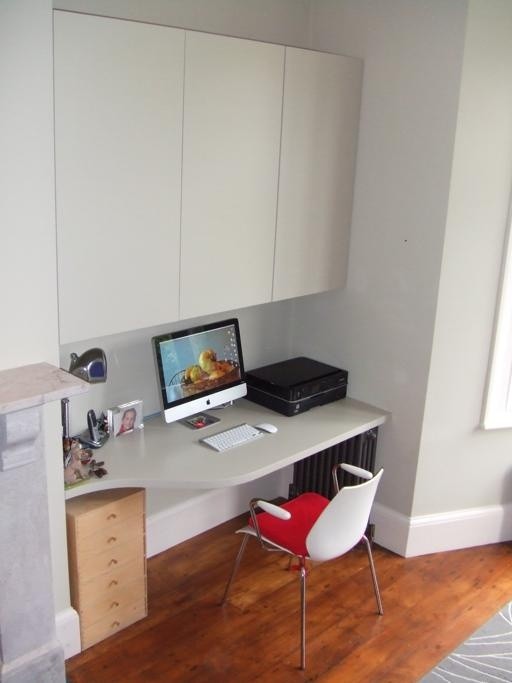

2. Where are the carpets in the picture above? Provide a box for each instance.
[412,599,512,683]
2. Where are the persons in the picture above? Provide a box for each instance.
[116,408,136,436]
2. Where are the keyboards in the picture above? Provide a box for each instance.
[199,423,264,452]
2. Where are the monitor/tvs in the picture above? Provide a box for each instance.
[151,317,250,429]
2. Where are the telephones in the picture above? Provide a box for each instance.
[78,409,109,449]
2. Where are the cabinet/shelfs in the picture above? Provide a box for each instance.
[53,8,188,345]
[184,30,288,322]
[65,489,152,655]
[272,42,363,308]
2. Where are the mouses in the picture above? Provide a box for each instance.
[255,423,279,434]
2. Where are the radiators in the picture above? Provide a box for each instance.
[287,421,378,546]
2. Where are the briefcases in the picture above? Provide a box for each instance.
[243,357,347,416]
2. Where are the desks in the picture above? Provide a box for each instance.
[64,397,391,489]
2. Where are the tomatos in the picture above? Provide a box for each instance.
[184,350,234,384]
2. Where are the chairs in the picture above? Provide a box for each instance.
[219,460,386,671]
